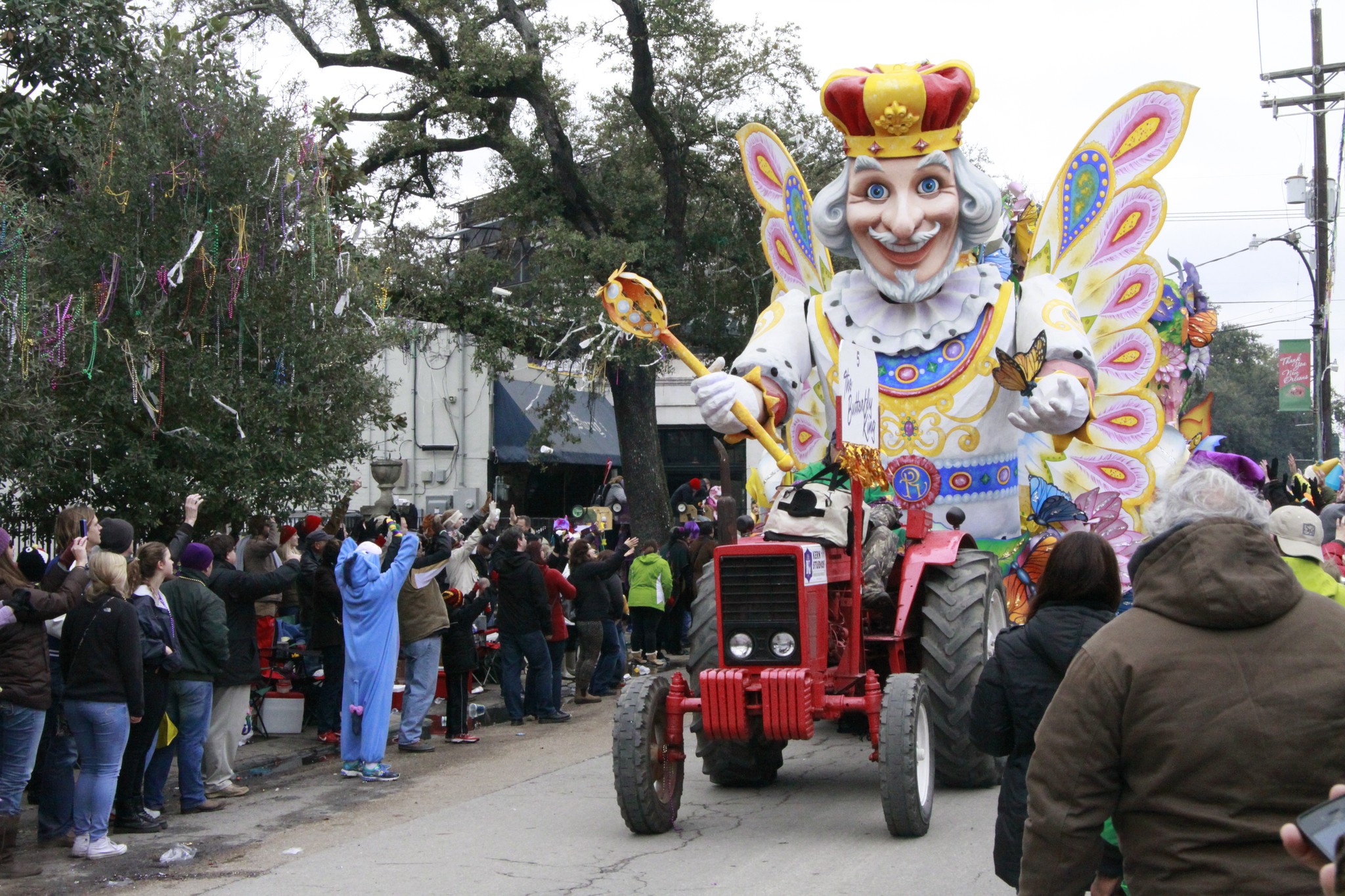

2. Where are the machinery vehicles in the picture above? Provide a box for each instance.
[612,507,1011,838]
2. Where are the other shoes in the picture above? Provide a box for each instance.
[41,781,249,846]
[398,738,435,753]
[862,591,895,611]
[510,709,571,726]
[572,680,626,703]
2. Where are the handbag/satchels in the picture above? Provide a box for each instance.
[762,464,870,546]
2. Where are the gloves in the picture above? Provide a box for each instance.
[484,500,501,524]
[3,588,33,620]
[1266,459,1318,502]
[491,570,499,580]
[472,583,481,592]
[350,502,447,539]
[552,529,569,555]
[479,491,493,514]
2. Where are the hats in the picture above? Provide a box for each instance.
[1272,504,1325,562]
[441,588,464,608]
[0,527,10,555]
[279,525,297,545]
[690,479,702,490]
[301,515,321,533]
[479,534,496,550]
[709,485,722,503]
[180,543,214,571]
[830,429,837,444]
[98,517,134,553]
[305,530,334,544]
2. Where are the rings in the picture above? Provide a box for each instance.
[78,544,83,546]
[1292,462,1294,463]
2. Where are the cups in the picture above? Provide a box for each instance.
[623,673,632,681]
[469,703,485,718]
[471,686,484,694]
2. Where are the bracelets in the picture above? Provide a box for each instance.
[1299,497,1308,503]
[74,565,85,568]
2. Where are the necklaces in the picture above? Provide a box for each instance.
[122,595,127,602]
[179,575,206,586]
[152,590,175,638]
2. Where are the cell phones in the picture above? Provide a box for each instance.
[1294,791,1344,863]
[80,520,88,538]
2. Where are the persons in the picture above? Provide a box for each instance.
[0,476,765,879]
[690,61,1201,629]
[971,454,1345,896]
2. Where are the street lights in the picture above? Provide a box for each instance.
[1246,235,1335,463]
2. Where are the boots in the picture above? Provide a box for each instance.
[631,649,664,666]
[1,811,44,878]
[561,652,576,680]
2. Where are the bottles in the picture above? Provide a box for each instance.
[636,665,650,674]
[441,716,447,726]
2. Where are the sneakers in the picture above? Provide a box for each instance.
[70,833,127,859]
[341,759,400,781]
[316,729,342,743]
[445,732,480,744]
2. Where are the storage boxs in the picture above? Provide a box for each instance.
[252,691,306,734]
[434,666,473,701]
[391,684,406,712]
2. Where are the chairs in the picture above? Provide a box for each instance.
[473,628,502,688]
[249,615,327,741]
